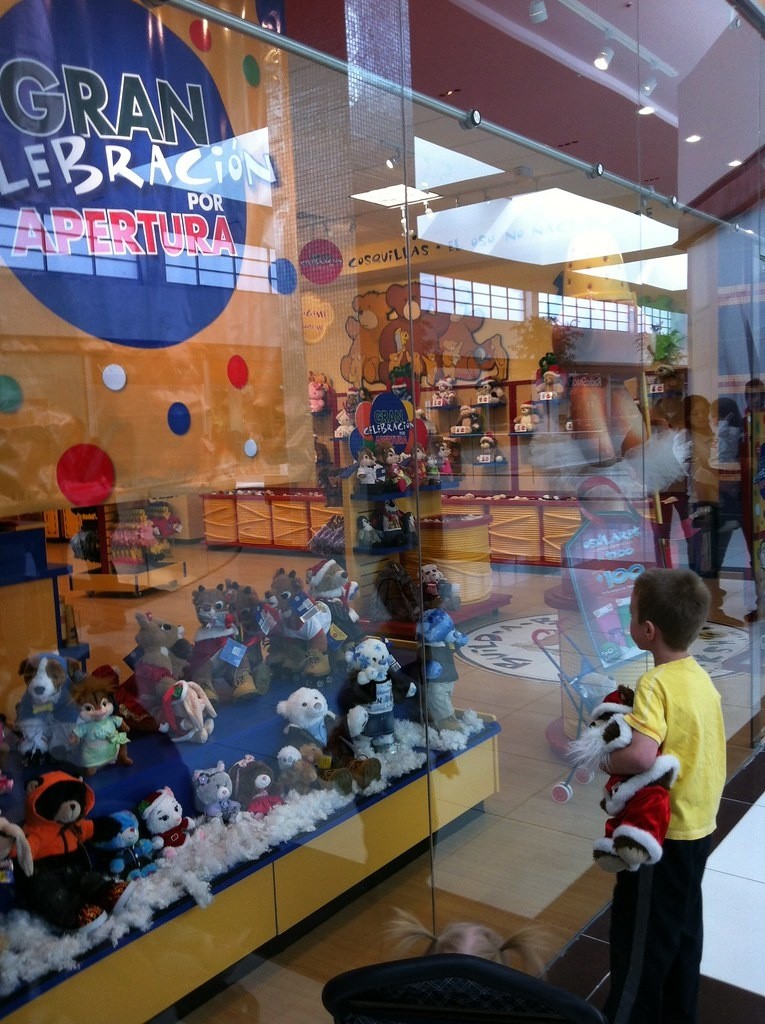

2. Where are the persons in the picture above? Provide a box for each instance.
[382,903,556,980]
[623,366,696,570]
[599,567,730,1024]
[708,397,743,533]
[673,394,745,627]
[739,377,765,621]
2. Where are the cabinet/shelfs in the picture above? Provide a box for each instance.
[1,626,501,1024]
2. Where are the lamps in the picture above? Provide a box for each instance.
[727,223,740,236]
[528,1,549,24]
[582,160,604,180]
[594,47,618,72]
[638,79,660,96]
[659,194,677,209]
[459,108,481,131]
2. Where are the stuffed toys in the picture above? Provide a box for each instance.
[568,683,679,874]
[1,364,565,938]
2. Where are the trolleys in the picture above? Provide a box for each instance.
[531,626,628,804]
